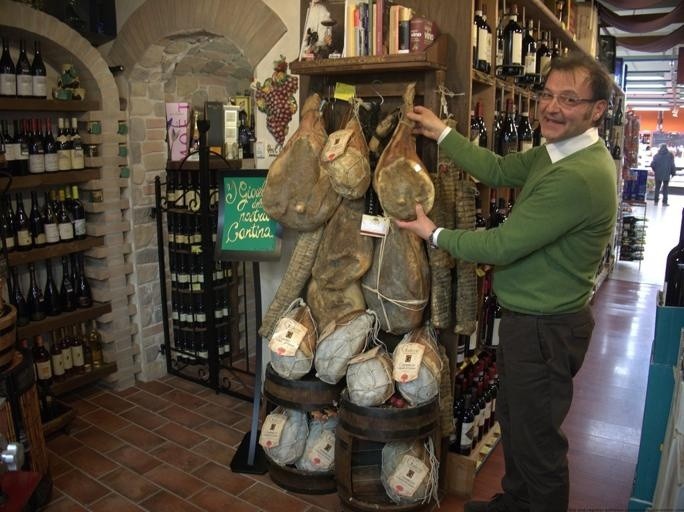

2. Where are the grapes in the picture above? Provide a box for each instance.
[256,77,298,146]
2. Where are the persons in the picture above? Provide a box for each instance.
[393,54,617,512]
[651,143,675,206]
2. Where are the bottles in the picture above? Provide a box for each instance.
[190,111,201,151]
[662,208,684,307]
[0,35,110,390]
[601,96,648,261]
[448,2,581,457]
[160,164,238,365]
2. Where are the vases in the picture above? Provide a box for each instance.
[0,303,17,372]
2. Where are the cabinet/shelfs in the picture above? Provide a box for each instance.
[0,100,118,396]
[167,160,244,371]
[290,0,626,500]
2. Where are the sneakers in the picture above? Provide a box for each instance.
[463,492,507,510]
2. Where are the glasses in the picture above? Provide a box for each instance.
[539,89,595,106]
